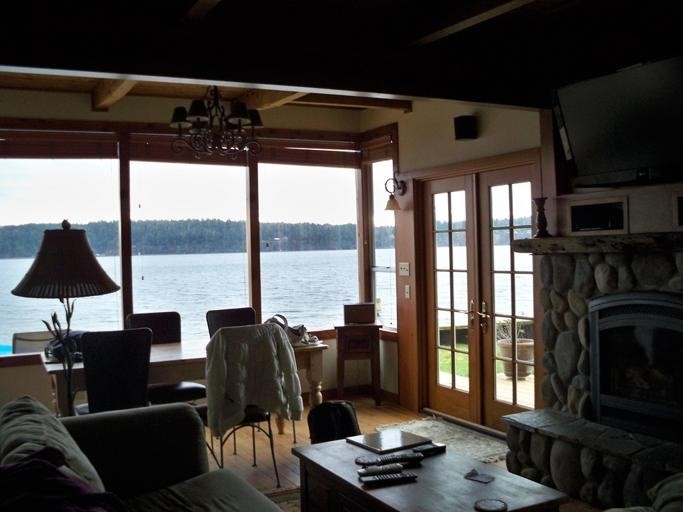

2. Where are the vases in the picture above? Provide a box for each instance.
[532,196,550,238]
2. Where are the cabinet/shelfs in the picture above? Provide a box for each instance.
[334,325,384,406]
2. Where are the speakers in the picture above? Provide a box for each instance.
[454,115,479,140]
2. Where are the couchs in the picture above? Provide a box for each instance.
[0,391,287,512]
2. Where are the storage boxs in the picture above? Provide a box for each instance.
[343,303,376,324]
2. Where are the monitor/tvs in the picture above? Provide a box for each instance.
[555,54,683,188]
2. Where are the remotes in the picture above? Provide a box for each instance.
[377,452,424,465]
[412,443,446,453]
[360,471,417,486]
[357,463,403,476]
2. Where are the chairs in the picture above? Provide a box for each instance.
[124,310,208,405]
[74,326,155,418]
[198,323,305,491]
[205,306,257,338]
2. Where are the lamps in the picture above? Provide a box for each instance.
[170,85,265,161]
[382,177,408,212]
[12,219,121,415]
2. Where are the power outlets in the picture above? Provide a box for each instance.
[398,262,411,277]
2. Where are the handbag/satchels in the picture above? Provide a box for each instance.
[264,314,310,348]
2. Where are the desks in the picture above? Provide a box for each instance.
[38,338,329,417]
